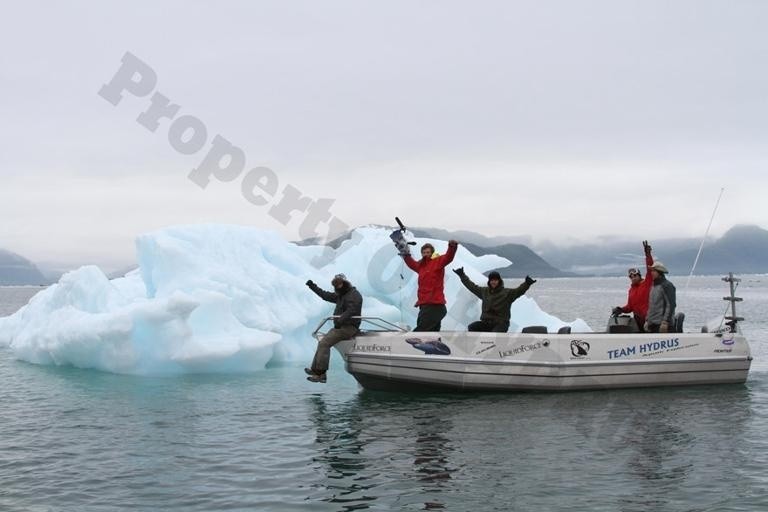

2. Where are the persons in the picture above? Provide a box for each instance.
[305,273,363,383]
[452,266,537,333]
[401,240,458,331]
[616,239,654,333]
[643,262,677,333]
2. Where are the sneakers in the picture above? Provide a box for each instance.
[304,368,315,374]
[308,375,326,383]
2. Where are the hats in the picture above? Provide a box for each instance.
[649,262,668,274]
[332,273,350,286]
[627,268,640,277]
[488,272,501,280]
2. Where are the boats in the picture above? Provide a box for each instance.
[310,268,756,390]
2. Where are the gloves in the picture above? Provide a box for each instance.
[306,280,313,286]
[613,307,623,314]
[337,316,344,324]
[643,240,651,254]
[660,321,668,332]
[644,322,648,330]
[453,267,464,275]
[526,275,536,284]
